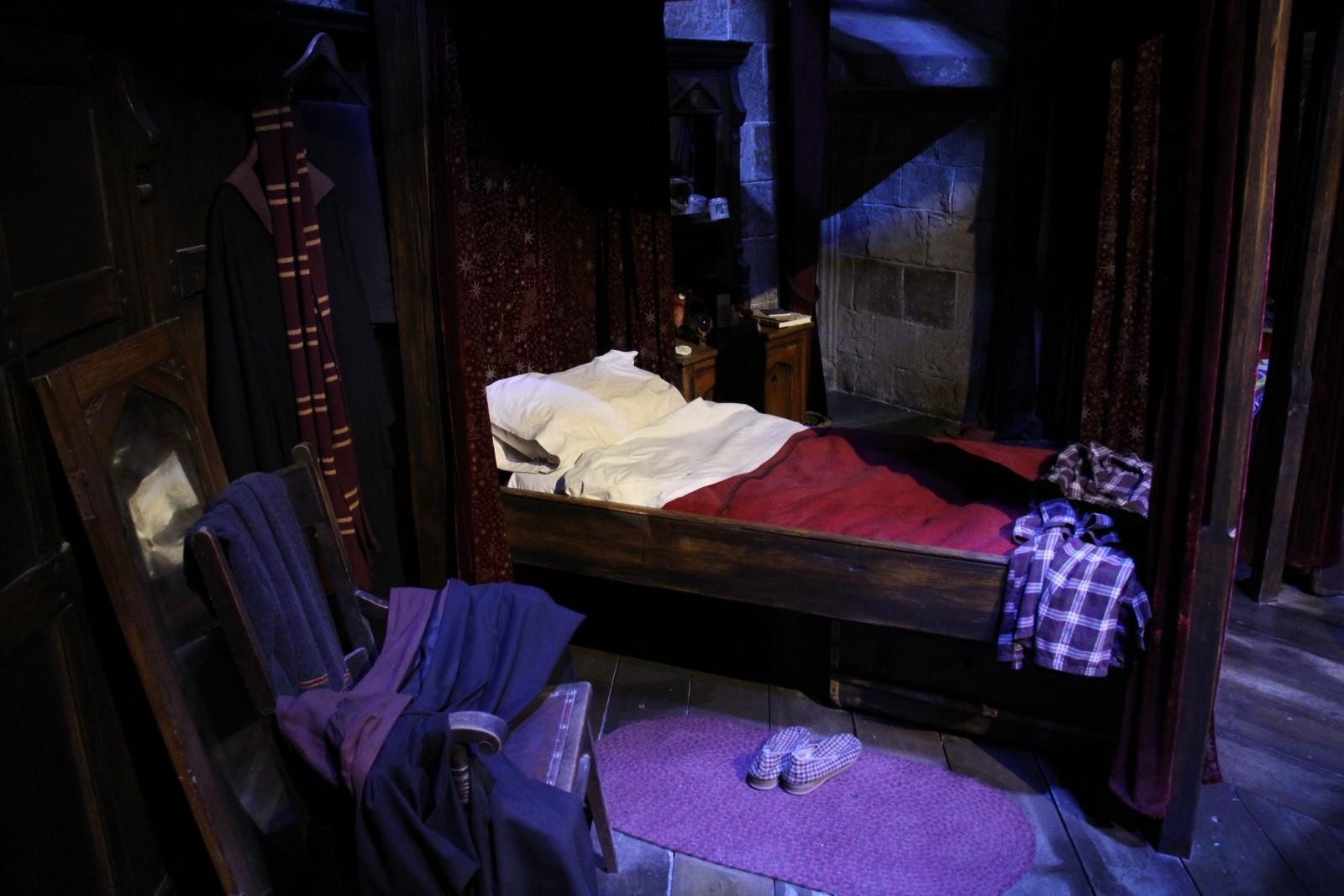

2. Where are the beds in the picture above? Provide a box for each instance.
[370,0,1294,861]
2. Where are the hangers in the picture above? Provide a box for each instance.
[206,138,334,219]
[282,0,373,110]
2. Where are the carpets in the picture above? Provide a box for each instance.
[588,716,1036,896]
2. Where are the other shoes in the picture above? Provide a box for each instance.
[746,725,810,790]
[781,731,863,797]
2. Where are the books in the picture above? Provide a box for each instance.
[752,309,812,329]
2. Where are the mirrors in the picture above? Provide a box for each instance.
[26,316,346,896]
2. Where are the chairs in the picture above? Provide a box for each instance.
[189,443,621,896]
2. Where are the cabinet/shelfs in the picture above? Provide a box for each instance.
[674,319,816,426]
